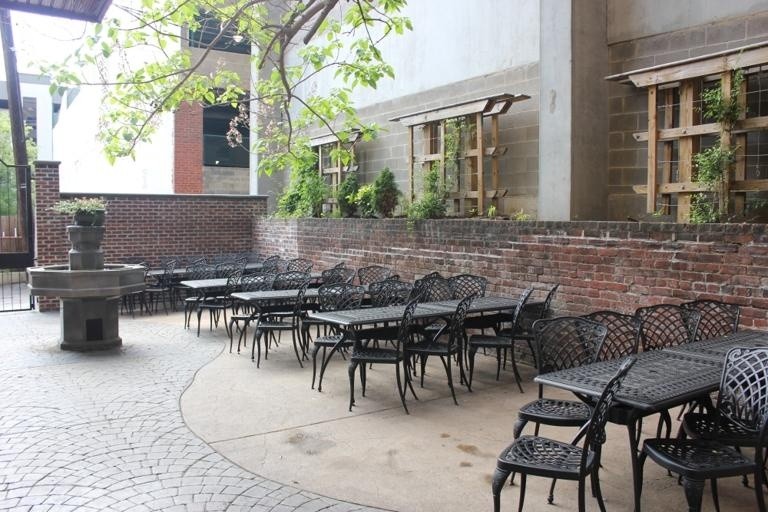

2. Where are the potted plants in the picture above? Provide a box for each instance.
[45,196,106,226]
